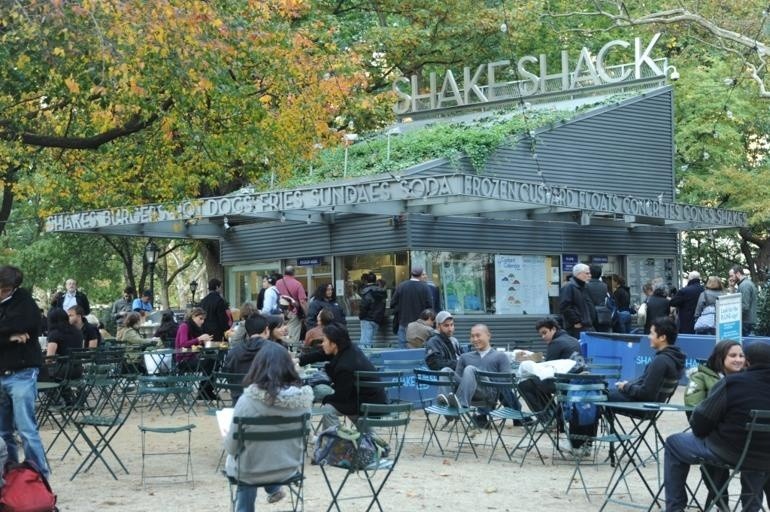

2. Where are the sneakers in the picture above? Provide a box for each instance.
[472,414,494,430]
[565,445,592,456]
[197,389,222,400]
[436,391,463,421]
[513,416,534,426]
[266,487,285,503]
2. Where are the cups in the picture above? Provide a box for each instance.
[38,336,47,349]
[497,347,505,352]
[145,320,159,326]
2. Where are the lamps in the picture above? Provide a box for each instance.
[222,214,231,231]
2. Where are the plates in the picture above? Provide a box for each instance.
[644,403,660,407]
[192,340,228,352]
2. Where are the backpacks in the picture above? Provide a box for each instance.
[270,287,299,320]
[563,370,605,436]
[0,459,60,512]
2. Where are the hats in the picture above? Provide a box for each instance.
[412,265,424,276]
[435,310,454,324]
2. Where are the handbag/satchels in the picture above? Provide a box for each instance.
[297,300,306,319]
[312,423,392,480]
[637,302,649,326]
[692,291,716,331]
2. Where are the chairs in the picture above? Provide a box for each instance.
[415,353,622,466]
[213,347,412,511]
[36,345,228,488]
[558,393,769,512]
